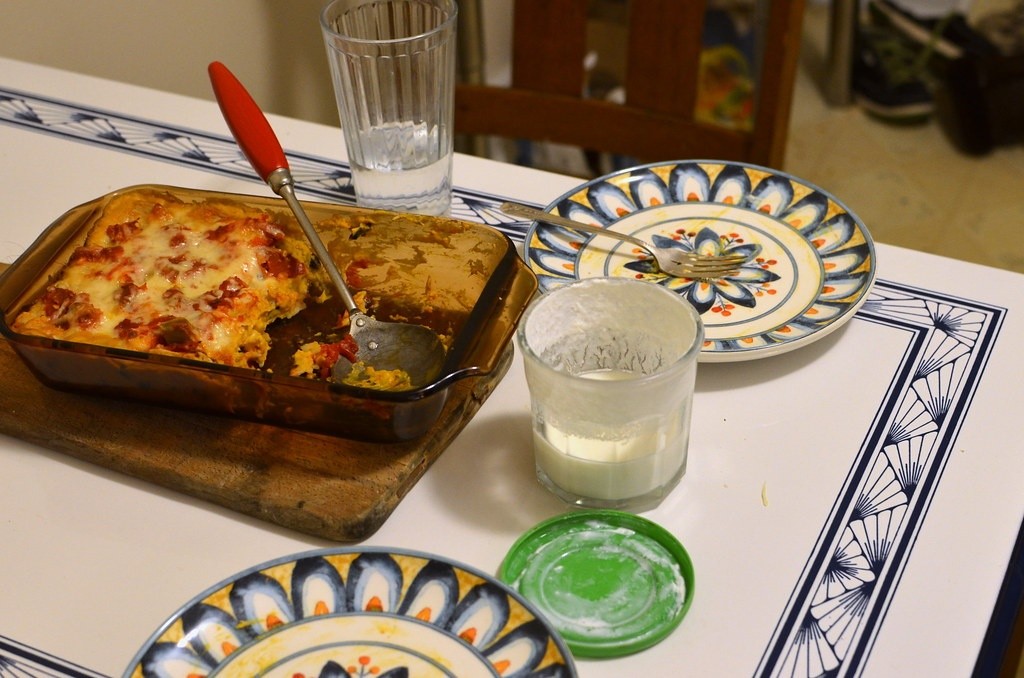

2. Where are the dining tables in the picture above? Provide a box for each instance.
[0,55,1024,678]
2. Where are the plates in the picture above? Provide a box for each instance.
[122,546,579,677]
[522,160,877,362]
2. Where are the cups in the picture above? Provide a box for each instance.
[319,0,458,216]
[518,277,705,515]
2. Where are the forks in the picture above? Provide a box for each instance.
[500,202,749,277]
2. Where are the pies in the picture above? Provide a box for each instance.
[9,187,323,369]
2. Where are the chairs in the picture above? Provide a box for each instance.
[455,0,801,171]
